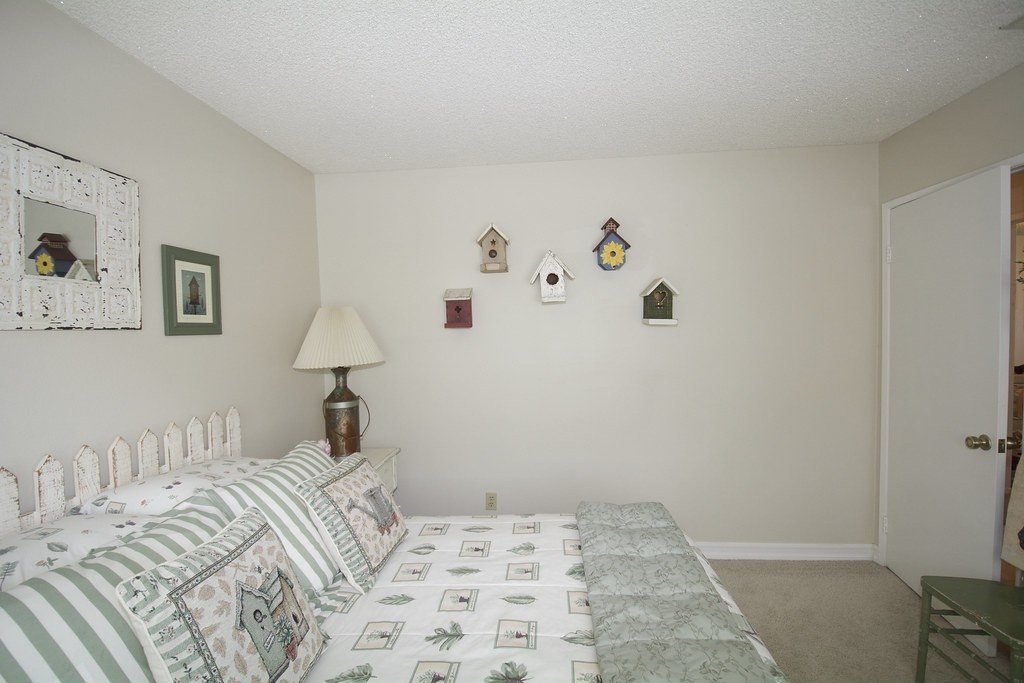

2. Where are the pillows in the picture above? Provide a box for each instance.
[294,452,414,595]
[0,515,158,594]
[71,456,275,515]
[0,493,232,683]
[115,506,333,683]
[203,440,344,600]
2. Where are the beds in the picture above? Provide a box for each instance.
[0,405,787,683]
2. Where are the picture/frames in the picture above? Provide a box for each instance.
[161,244,222,336]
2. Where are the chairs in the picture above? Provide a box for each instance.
[915,456,1024,683]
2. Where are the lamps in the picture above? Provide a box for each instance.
[292,305,386,461]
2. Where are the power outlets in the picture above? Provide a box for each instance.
[485,492,497,510]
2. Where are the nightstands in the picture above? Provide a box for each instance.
[361,446,402,496]
